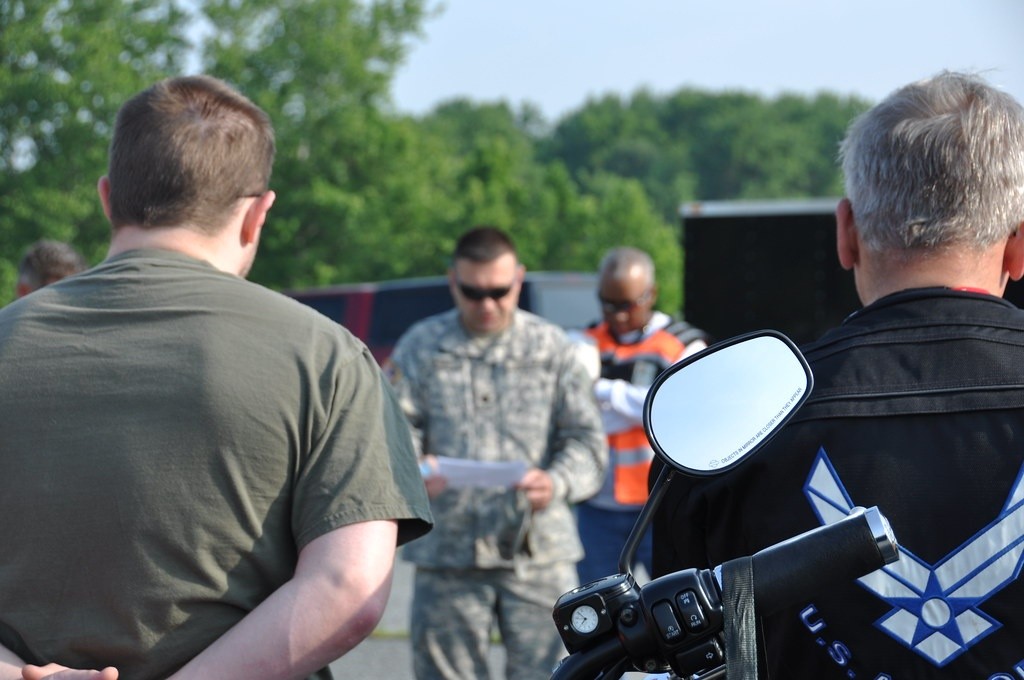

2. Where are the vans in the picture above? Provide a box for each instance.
[281,268,612,365]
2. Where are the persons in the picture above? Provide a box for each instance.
[384,226,611,680]
[647,67,1023,680]
[0,75,436,680]
[564,248,708,585]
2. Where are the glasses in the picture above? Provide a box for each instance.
[598,288,652,312]
[454,273,515,301]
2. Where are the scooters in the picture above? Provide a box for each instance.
[538,328,902,680]
